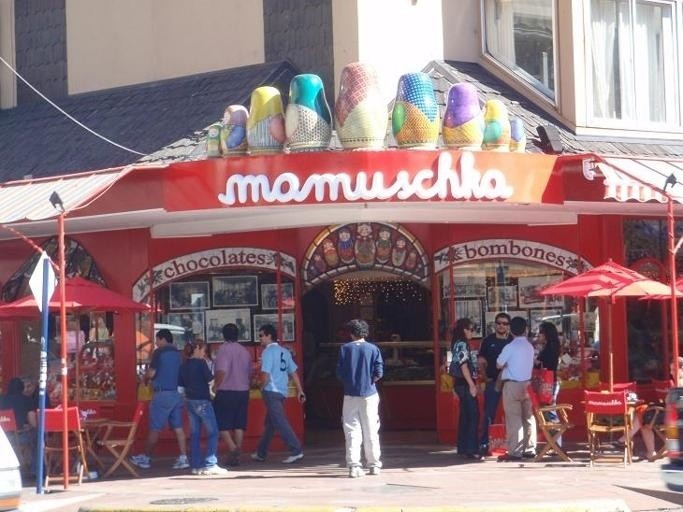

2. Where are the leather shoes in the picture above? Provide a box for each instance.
[498,454,521,460]
[523,454,535,458]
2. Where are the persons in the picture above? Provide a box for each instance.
[21,315,112,368]
[129,328,189,468]
[495,317,536,461]
[0,378,37,478]
[535,323,562,455]
[669,357,682,389]
[614,355,682,460]
[214,323,250,467]
[479,312,514,453]
[177,340,227,476]
[334,319,384,478]
[448,317,484,460]
[21,375,49,409]
[251,326,306,463]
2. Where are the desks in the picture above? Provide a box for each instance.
[50,415,112,481]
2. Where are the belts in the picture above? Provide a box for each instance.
[154,389,174,391]
[504,379,517,382]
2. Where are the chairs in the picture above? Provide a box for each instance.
[95,401,147,482]
[523,374,678,469]
[0,407,35,478]
[34,406,92,491]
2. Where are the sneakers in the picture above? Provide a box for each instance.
[128,453,151,469]
[460,452,486,461]
[282,454,304,463]
[611,440,626,448]
[202,464,228,475]
[191,467,203,474]
[251,453,265,462]
[232,459,240,467]
[637,455,650,460]
[171,455,191,470]
[221,451,240,465]
[478,447,492,455]
[546,449,567,456]
[349,466,365,477]
[370,467,380,475]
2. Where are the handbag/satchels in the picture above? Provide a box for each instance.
[531,357,555,405]
[449,359,473,378]
[644,404,665,424]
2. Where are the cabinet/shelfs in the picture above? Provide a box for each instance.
[309,339,436,433]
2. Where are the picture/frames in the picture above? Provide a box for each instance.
[166,274,298,351]
[440,268,567,340]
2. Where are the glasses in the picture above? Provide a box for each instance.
[468,328,474,331]
[259,334,264,337]
[496,321,509,325]
[539,329,545,333]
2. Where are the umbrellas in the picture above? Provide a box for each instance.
[0,274,148,418]
[637,274,682,303]
[536,258,682,442]
